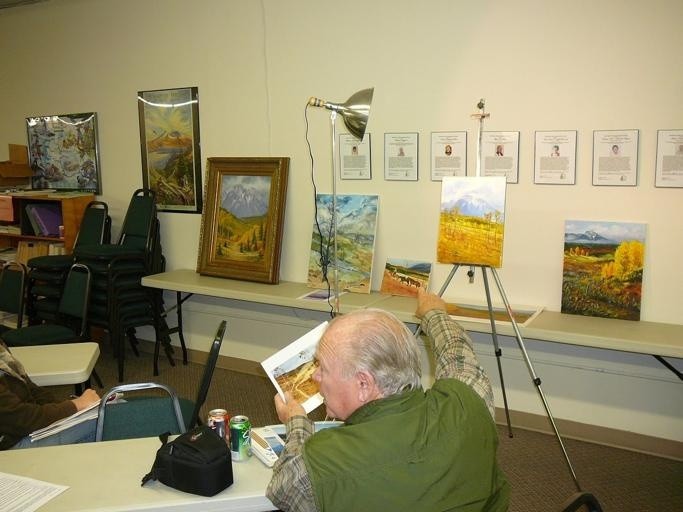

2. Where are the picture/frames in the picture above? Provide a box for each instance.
[446,301,545,328]
[24,111,104,195]
[137,86,202,214]
[195,156,291,285]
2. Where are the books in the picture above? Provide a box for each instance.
[28,393,129,442]
[249,419,345,468]
[1,203,65,272]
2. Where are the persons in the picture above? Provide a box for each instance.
[612,144,618,154]
[352,146,358,156]
[0,336,128,451]
[399,147,404,156]
[675,145,683,154]
[495,145,502,155]
[445,145,453,155]
[264,285,512,511]
[551,145,559,155]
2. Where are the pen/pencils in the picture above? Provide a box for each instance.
[69,395,79,399]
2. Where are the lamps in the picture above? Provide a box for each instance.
[304,87,375,318]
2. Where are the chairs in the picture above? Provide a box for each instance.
[95,382,187,442]
[0,187,177,397]
[124,320,227,432]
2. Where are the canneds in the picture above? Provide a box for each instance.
[206,409,228,442]
[227,415,252,464]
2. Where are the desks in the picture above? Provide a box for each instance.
[365,294,683,382]
[0,424,281,512]
[140,268,393,377]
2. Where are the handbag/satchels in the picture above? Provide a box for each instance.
[140,421,236,498]
